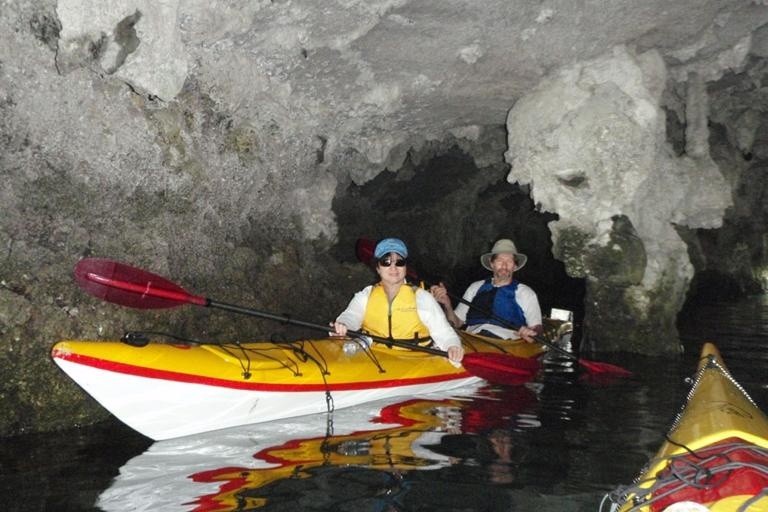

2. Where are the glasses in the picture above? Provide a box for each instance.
[379,259,406,267]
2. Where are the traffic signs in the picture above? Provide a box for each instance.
[611,337,768,512]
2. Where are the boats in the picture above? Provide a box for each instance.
[48,303,574,441]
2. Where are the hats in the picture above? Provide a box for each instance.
[480,239,528,272]
[374,238,408,259]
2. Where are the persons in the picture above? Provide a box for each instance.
[327,236,466,364]
[430,239,544,343]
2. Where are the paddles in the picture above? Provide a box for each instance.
[358,239,635,378]
[74,256,549,388]
